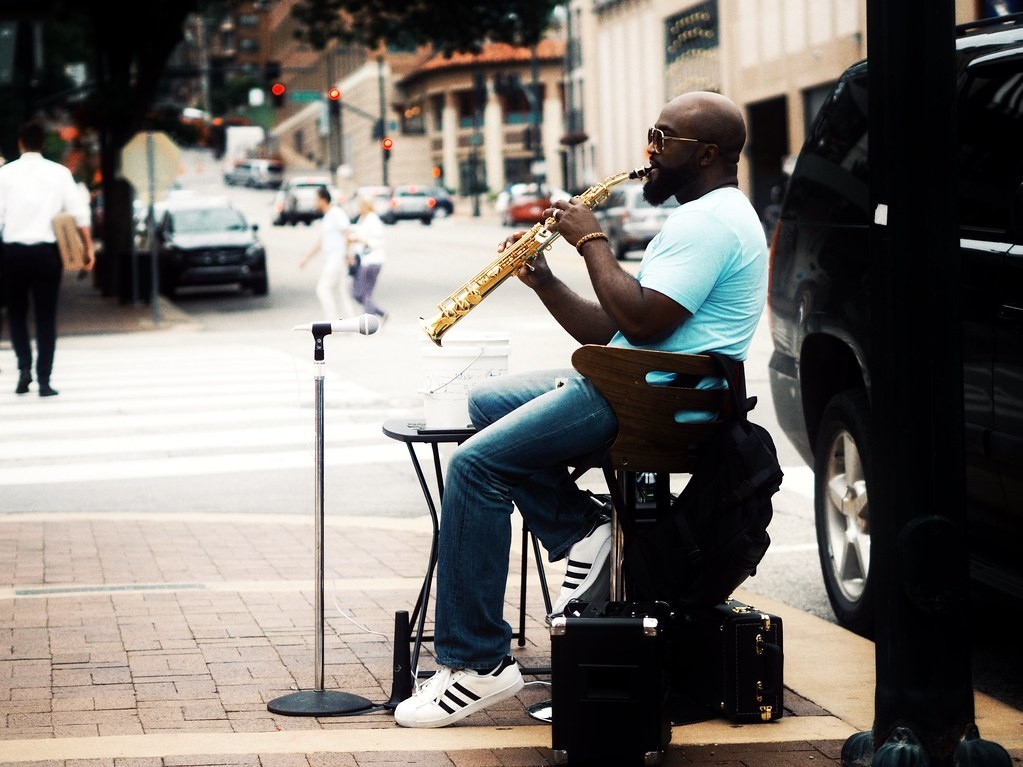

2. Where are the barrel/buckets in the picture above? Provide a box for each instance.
[418,337,510,429]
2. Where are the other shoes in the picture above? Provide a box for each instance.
[39,386,58,396]
[16,372,33,393]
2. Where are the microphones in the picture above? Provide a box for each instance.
[293,314,379,335]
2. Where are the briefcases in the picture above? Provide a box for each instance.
[714,598,784,722]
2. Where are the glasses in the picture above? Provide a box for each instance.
[648,127,723,155]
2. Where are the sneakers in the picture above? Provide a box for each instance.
[394,654,525,729]
[553,515,613,616]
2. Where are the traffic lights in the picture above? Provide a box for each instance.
[432,166,443,179]
[382,137,392,160]
[271,83,286,109]
[329,88,341,116]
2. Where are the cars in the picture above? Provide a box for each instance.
[153,206,267,303]
[767,0,1022,696]
[346,186,453,224]
[274,177,333,225]
[222,156,284,188]
[595,183,683,258]
[498,184,551,223]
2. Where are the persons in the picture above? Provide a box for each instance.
[396,92,769,732]
[0,123,94,397]
[298,187,389,331]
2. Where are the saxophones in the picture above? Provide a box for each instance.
[414,165,655,348]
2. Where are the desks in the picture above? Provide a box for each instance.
[382,419,552,689]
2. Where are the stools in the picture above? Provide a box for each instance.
[528,344,747,724]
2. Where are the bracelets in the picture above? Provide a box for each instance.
[576,232,608,256]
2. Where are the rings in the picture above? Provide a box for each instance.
[552,209,560,217]
